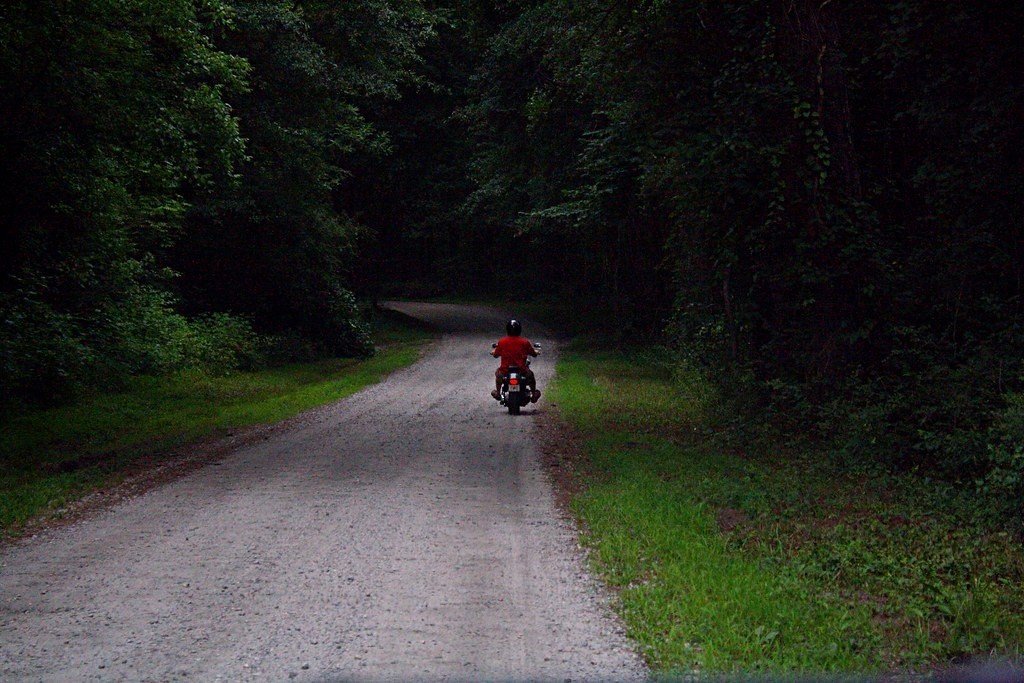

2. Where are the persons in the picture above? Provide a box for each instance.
[491,319,541,403]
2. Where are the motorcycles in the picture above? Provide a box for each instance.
[490,341,543,414]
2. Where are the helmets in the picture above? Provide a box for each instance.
[506,319,522,336]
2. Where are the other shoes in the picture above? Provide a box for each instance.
[491,390,501,401]
[531,390,541,403]
